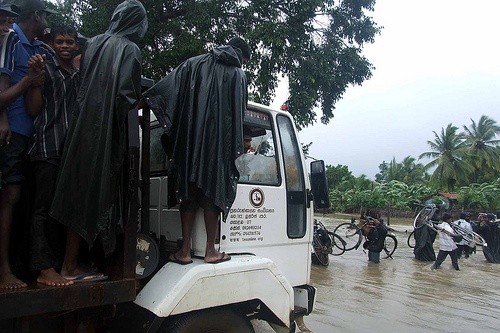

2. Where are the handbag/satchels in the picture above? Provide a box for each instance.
[363,240,375,249]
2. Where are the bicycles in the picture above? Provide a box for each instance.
[330,216,398,261]
[407,202,489,249]
[313,212,346,266]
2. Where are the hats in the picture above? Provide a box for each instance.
[13,0,58,17]
[0,0,19,18]
[227,37,250,61]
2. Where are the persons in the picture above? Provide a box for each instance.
[243,125,256,154]
[414,205,500,271]
[363,216,388,264]
[166,37,250,265]
[0,0,88,288]
[51,1,148,282]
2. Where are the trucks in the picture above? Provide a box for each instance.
[1,100,331,333]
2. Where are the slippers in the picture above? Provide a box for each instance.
[70,270,108,283]
[167,252,193,265]
[208,252,230,264]
